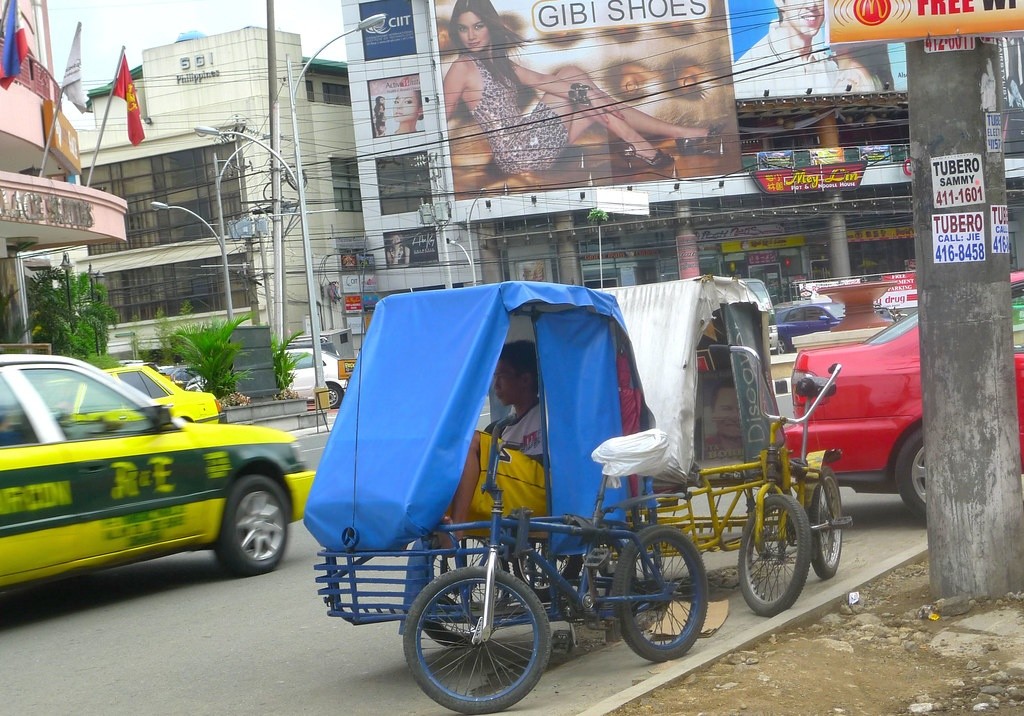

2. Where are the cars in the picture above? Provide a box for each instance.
[39,332,350,440]
[791,271,1024,527]
[739,278,846,355]
[0,354,318,602]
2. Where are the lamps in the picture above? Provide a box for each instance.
[486,200,491,207]
[580,192,585,199]
[846,85,852,91]
[675,183,679,189]
[805,88,812,94]
[763,89,769,96]
[719,180,725,186]
[884,82,889,90]
[531,196,536,204]
[628,186,632,190]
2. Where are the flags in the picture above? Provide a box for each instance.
[112,52,145,146]
[61,22,87,114]
[0,0,28,90]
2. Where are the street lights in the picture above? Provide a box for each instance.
[151,131,282,326]
[193,12,387,410]
[450,187,487,286]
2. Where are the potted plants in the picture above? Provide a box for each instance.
[587,209,609,225]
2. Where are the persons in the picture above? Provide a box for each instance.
[704,380,744,459]
[800,283,819,298]
[373,96,387,137]
[732,0,894,99]
[393,90,424,134]
[387,235,410,268]
[444,0,730,174]
[438,340,547,558]
[50,380,76,412]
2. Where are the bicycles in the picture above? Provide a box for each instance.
[404,415,709,714]
[709,342,852,617]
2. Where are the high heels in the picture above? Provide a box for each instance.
[675,123,726,155]
[622,142,674,168]
